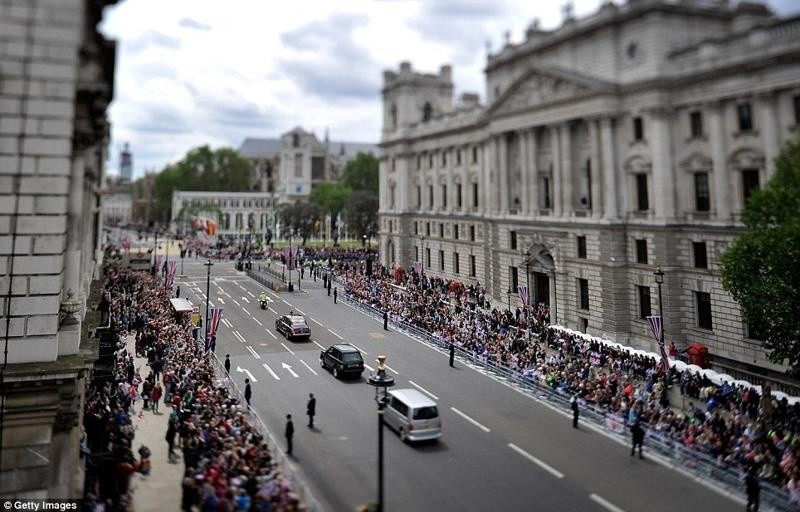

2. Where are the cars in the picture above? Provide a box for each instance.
[275,314,311,340]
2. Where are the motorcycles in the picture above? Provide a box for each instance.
[258,300,267,310]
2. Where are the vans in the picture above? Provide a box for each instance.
[377,387,442,444]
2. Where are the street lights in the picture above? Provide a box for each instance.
[506,286,512,313]
[654,265,671,407]
[202,260,215,353]
[365,354,395,511]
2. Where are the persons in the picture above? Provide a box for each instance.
[259,291,272,301]
[309,267,313,279]
[339,261,581,402]
[176,229,382,273]
[82,375,301,512]
[301,267,305,279]
[324,274,326,290]
[327,277,332,297]
[333,286,338,304]
[224,354,231,370]
[306,392,316,428]
[100,235,211,378]
[742,460,761,510]
[313,269,318,281]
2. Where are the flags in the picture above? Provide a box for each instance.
[205,304,221,355]
[189,215,219,233]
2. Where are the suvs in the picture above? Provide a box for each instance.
[320,343,365,379]
